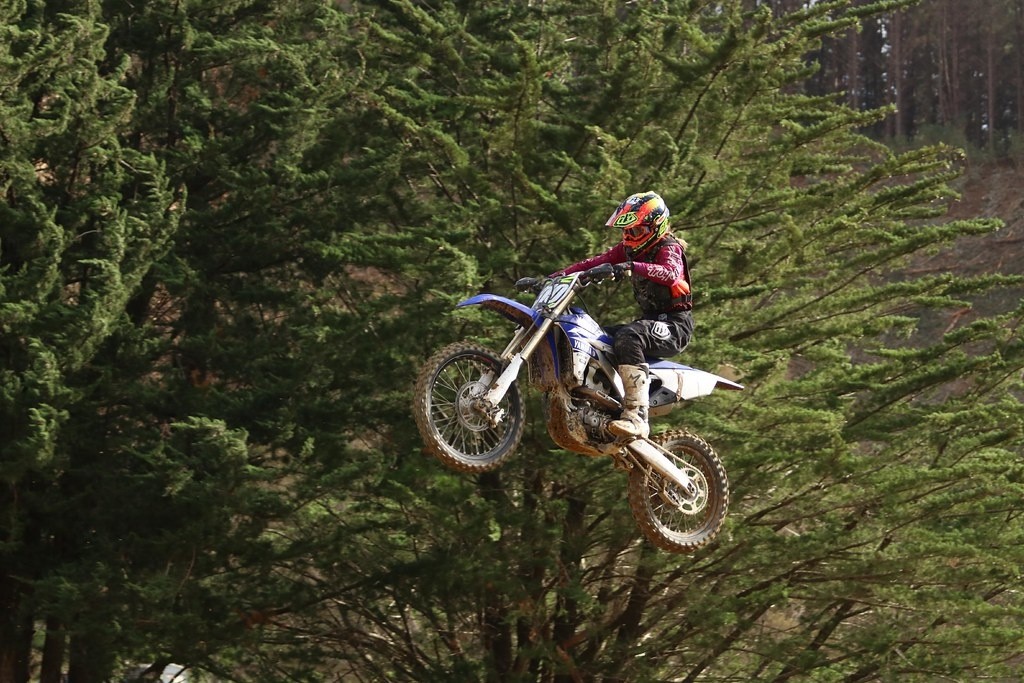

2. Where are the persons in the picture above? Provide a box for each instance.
[532,191,694,438]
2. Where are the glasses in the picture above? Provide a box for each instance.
[623,205,669,240]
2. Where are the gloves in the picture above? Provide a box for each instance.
[516,275,548,296]
[583,262,627,283]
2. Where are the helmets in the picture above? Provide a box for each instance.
[605,190,670,261]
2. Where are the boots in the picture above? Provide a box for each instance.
[608,363,650,437]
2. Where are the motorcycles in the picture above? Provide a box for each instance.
[411,262,744,554]
